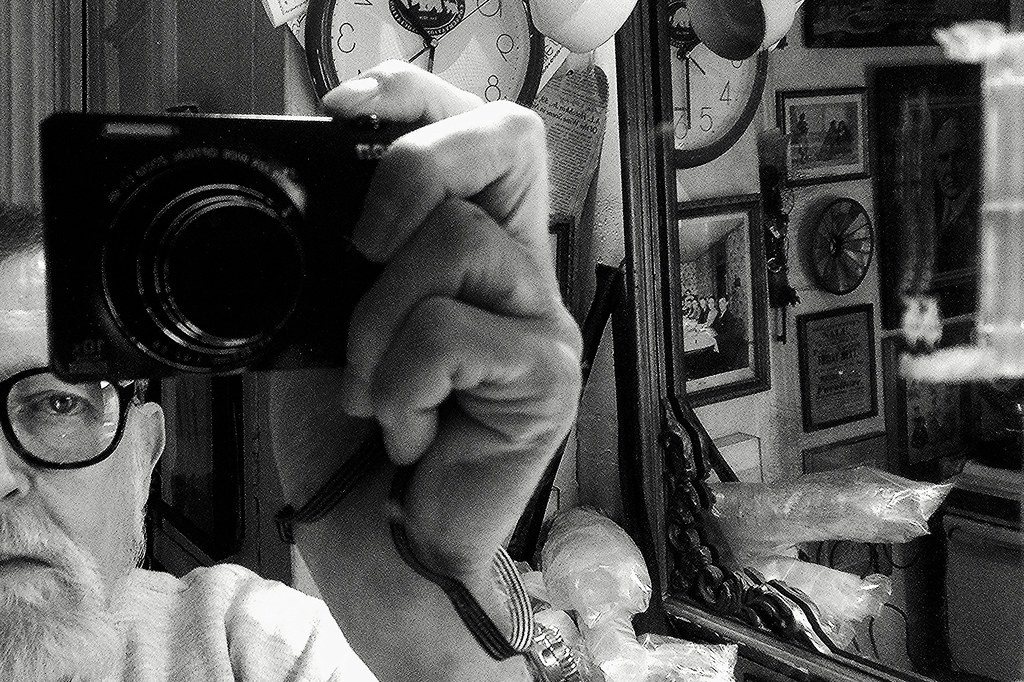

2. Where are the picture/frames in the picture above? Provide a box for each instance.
[796,302,878,433]
[804,0,1012,50]
[803,430,892,579]
[895,336,973,476]
[548,215,574,315]
[678,194,772,408]
[775,85,873,187]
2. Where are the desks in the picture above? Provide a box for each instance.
[682,319,717,354]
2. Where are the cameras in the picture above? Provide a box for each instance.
[39,111,430,380]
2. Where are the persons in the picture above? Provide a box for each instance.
[0,60,583,682]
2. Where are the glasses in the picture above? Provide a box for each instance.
[0,366,138,470]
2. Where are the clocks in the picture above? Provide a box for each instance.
[668,0,770,169]
[305,0,545,109]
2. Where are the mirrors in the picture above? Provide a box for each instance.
[614,2,1024,681]
